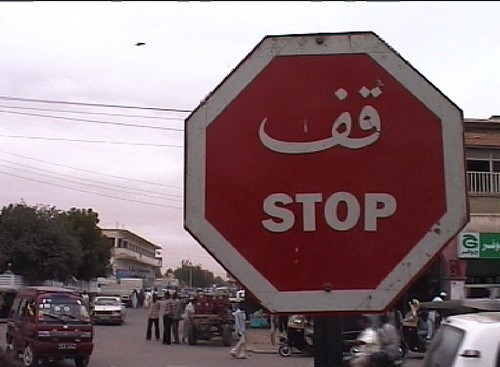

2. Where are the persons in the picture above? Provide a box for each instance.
[81,290,91,312]
[172,292,186,342]
[402,299,420,351]
[376,315,399,361]
[230,301,248,359]
[145,294,161,340]
[305,316,314,346]
[182,299,196,344]
[162,291,176,344]
[385,301,405,356]
[426,290,448,340]
[348,316,382,367]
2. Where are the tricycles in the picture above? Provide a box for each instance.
[186,293,235,345]
[278,319,314,357]
[396,301,464,357]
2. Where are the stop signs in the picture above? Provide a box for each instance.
[184,32,471,314]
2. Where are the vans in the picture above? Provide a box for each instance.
[7,286,92,367]
[421,313,500,367]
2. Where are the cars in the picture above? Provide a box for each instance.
[90,296,126,324]
[155,289,175,299]
